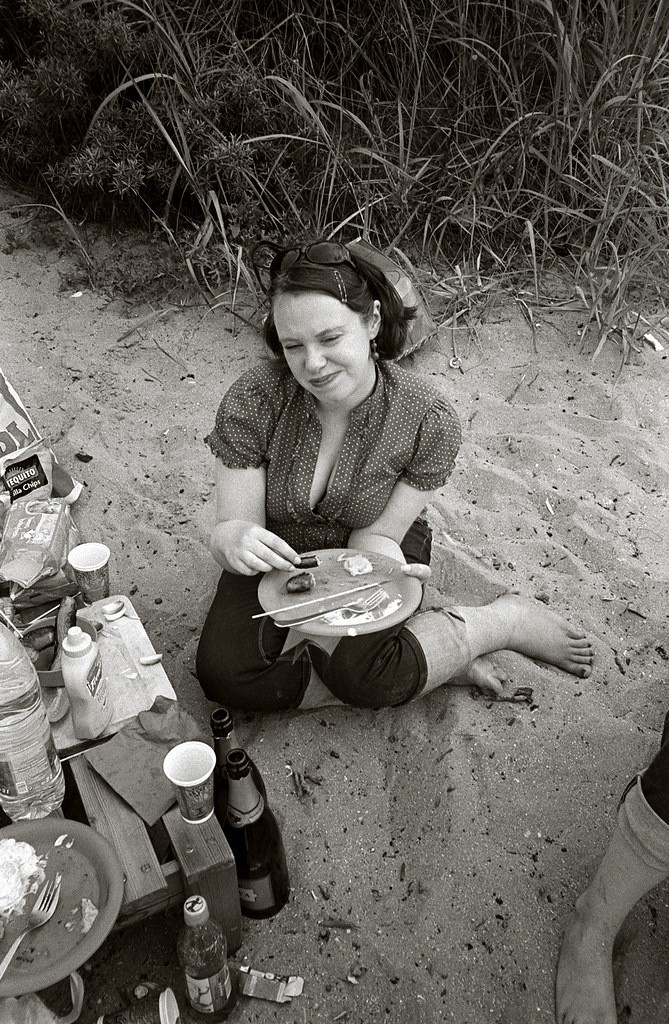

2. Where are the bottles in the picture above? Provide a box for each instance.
[59,626,114,740]
[209,707,268,841]
[222,748,290,920]
[0,621,67,823]
[175,895,237,1021]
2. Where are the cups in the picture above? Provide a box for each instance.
[163,740,217,824]
[67,542,111,608]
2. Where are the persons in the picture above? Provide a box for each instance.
[194,241,596,712]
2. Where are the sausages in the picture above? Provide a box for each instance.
[286,572,313,593]
[19,624,56,663]
[293,555,320,568]
[48,596,77,671]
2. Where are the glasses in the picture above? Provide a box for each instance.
[278,242,362,274]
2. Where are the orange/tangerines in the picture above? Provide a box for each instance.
[46,688,69,721]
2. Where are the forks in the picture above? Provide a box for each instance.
[274,586,389,627]
[0,880,62,981]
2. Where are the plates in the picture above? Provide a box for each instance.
[258,547,424,635]
[0,818,125,997]
[96,986,180,1024]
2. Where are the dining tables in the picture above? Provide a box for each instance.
[13,593,245,956]
